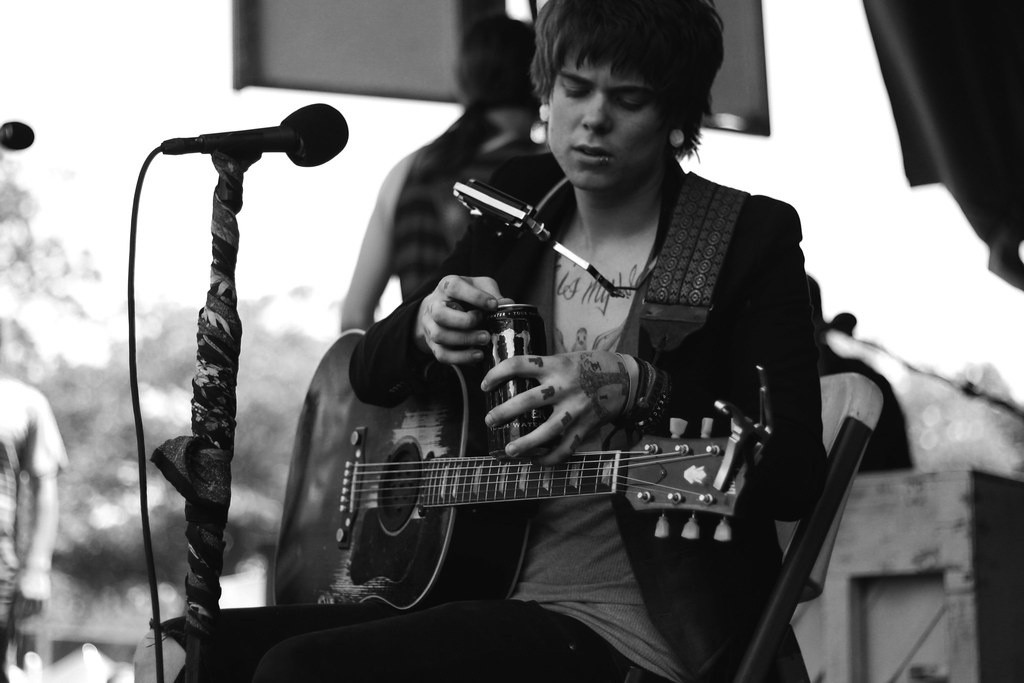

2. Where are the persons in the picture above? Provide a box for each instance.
[0,375,70,683]
[135,0,829,683]
[340,17,552,331]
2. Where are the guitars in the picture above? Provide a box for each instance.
[274,323,758,618]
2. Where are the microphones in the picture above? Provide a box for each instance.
[158,102,347,168]
[0,121,34,149]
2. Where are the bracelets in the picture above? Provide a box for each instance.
[601,352,671,451]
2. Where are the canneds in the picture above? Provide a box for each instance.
[476,303,560,461]
